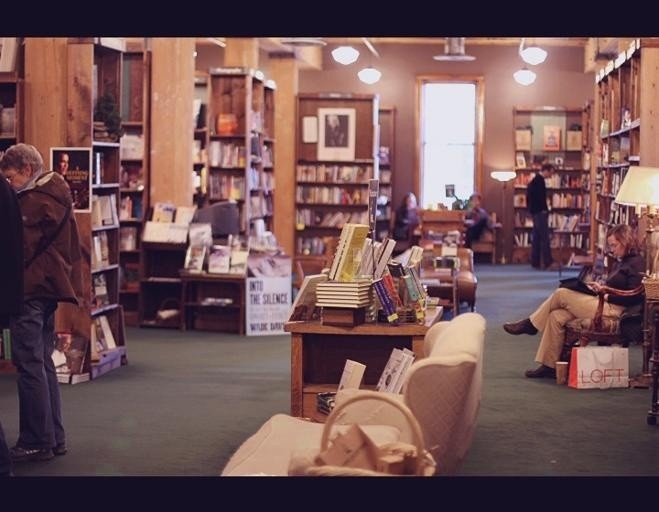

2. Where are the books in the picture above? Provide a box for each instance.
[263,196,274,216]
[1,104,16,134]
[379,186,392,202]
[295,236,333,255]
[93,314,117,351]
[250,196,261,220]
[200,295,233,307]
[209,140,246,168]
[373,246,439,326]
[600,108,632,137]
[514,192,590,209]
[251,219,277,252]
[120,165,144,189]
[262,143,274,167]
[287,274,328,321]
[0,38,18,73]
[250,110,264,132]
[208,173,245,200]
[251,167,262,188]
[120,132,145,159]
[378,168,392,183]
[297,165,375,184]
[379,146,392,164]
[92,232,109,270]
[90,273,110,310]
[513,231,590,249]
[194,141,206,163]
[140,202,194,243]
[377,206,391,221]
[93,151,104,185]
[596,137,631,166]
[594,200,630,227]
[328,222,396,281]
[3,329,11,359]
[193,167,206,194]
[184,223,252,275]
[296,185,369,206]
[315,278,372,309]
[433,246,460,272]
[120,197,134,219]
[264,171,275,192]
[296,208,370,229]
[93,121,118,142]
[596,168,629,196]
[335,359,367,393]
[515,211,591,231]
[516,172,592,190]
[250,133,262,163]
[597,224,609,250]
[90,193,120,229]
[118,227,137,251]
[375,349,416,396]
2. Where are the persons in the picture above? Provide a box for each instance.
[327,115,346,146]
[527,164,561,271]
[49,334,72,372]
[462,194,490,248]
[53,151,76,209]
[392,192,420,240]
[0,142,83,464]
[503,224,645,380]
[0,171,26,479]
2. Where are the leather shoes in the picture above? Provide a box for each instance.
[504,318,538,335]
[525,364,556,379]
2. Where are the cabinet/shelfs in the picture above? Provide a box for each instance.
[513,100,597,263]
[589,38,659,262]
[284,304,444,422]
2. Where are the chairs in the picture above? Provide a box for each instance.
[572,256,593,280]
[417,246,478,312]
[559,212,659,378]
[221,311,488,476]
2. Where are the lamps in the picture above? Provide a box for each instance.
[490,171,516,263]
[432,38,475,61]
[616,166,659,391]
[515,36,546,85]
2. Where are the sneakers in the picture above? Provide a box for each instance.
[531,264,545,271]
[8,444,54,464]
[16,440,68,455]
[544,262,561,271]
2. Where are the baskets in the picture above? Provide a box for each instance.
[287,391,438,479]
[640,278,659,300]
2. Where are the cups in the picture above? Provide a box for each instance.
[555,361,569,385]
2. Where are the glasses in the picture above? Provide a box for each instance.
[607,242,621,250]
[5,167,23,184]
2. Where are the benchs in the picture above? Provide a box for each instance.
[379,210,497,263]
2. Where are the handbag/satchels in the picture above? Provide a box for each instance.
[568,346,629,390]
[559,280,593,296]
